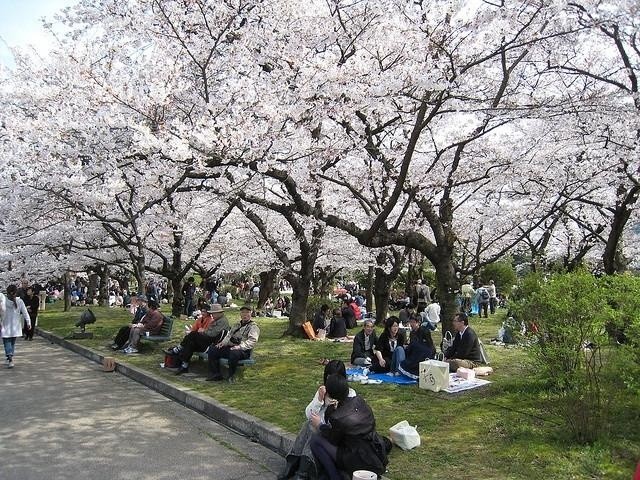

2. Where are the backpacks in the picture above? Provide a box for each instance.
[481,289,489,299]
[419,288,425,299]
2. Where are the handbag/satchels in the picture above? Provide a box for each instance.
[318,329,326,338]
[419,359,450,392]
[442,331,455,350]
[303,321,315,339]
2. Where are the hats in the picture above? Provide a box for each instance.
[240,306,251,310]
[137,295,148,302]
[207,304,225,313]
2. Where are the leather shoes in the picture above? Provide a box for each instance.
[277,463,293,479]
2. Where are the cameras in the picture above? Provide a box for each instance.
[230,336,242,345]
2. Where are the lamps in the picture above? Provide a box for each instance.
[71,307,97,339]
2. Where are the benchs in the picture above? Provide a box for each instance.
[191,346,258,384]
[140,318,174,349]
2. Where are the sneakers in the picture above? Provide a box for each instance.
[175,366,188,375]
[205,375,223,381]
[124,346,138,353]
[162,348,179,356]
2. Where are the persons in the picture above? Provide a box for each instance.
[310,372,389,480]
[266,288,292,316]
[278,360,357,480]
[388,279,497,317]
[110,294,150,350]
[0,292,6,327]
[312,284,367,339]
[207,306,260,381]
[161,303,229,376]
[124,300,164,353]
[50,279,262,317]
[16,279,30,338]
[350,313,489,380]
[3,285,32,368]
[24,288,40,340]
